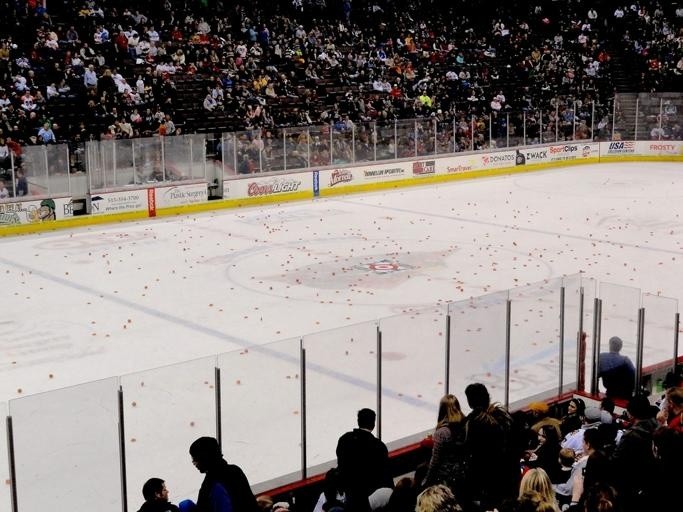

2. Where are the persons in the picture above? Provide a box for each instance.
[596,333,640,401]
[302,369,682,512]
[1,0,683,199]
[135,476,178,511]
[187,436,260,512]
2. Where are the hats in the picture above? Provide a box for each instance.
[627,395,656,420]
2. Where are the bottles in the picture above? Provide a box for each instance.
[655,379,663,393]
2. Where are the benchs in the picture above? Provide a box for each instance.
[163,74,242,132]
[268,79,360,116]
